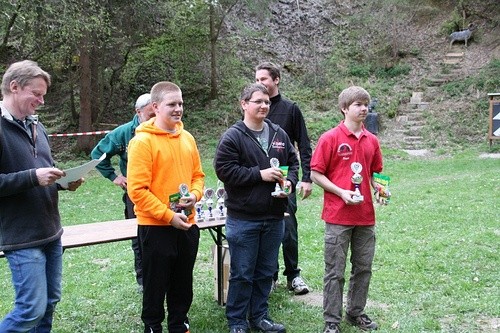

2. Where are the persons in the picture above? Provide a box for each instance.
[241,63,312,296]
[126,82,205,333]
[310,87,390,333]
[89,93,157,287]
[0,61,86,333]
[214,85,300,333]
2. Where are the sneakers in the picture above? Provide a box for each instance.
[229,324,249,333]
[345,311,376,331]
[247,315,286,333]
[287,276,309,295]
[323,322,341,333]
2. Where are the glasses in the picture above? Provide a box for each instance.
[249,100,271,106]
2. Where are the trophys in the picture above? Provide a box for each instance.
[179,184,189,215]
[351,162,364,201]
[195,187,227,223]
[270,157,284,196]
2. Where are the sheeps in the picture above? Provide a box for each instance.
[449,23,480,48]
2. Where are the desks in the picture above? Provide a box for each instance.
[61,207,290,306]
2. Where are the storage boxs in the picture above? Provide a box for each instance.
[211,245,230,303]
